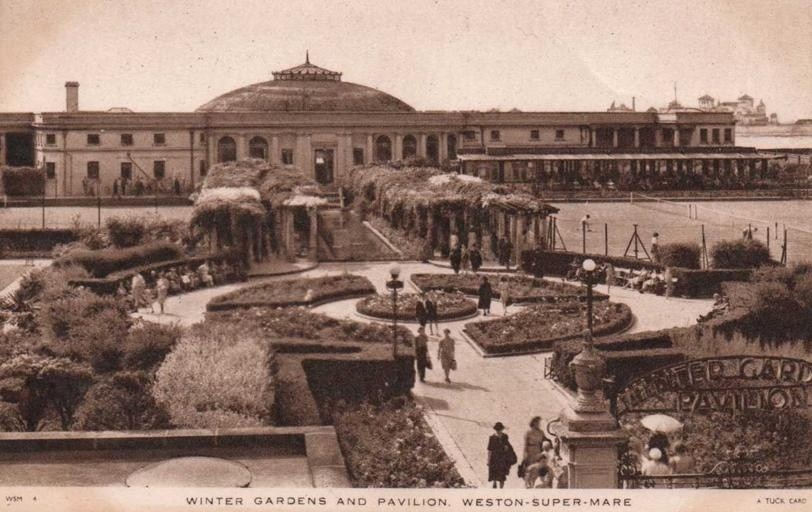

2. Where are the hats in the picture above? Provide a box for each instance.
[492,423,505,429]
[649,448,661,459]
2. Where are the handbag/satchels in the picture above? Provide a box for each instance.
[510,451,516,464]
[518,460,527,477]
[426,357,433,369]
[451,360,456,370]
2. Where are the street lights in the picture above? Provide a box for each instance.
[385,260,404,357]
[581,257,597,341]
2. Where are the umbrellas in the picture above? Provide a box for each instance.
[638,413,684,432]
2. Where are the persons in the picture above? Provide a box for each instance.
[117,257,241,315]
[447,228,664,296]
[414,294,428,334]
[695,294,730,321]
[478,276,493,315]
[425,289,443,337]
[81,174,180,198]
[574,214,595,233]
[712,292,721,310]
[415,326,433,383]
[742,226,758,241]
[435,329,456,384]
[497,274,511,315]
[486,416,692,488]
